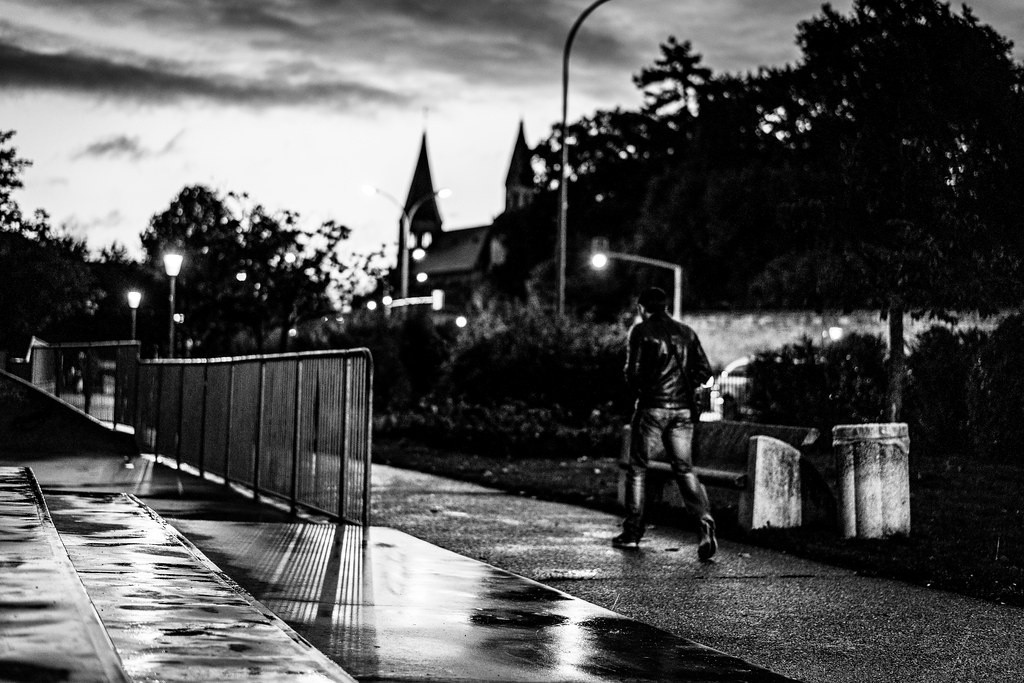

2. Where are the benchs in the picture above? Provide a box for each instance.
[618,422,820,531]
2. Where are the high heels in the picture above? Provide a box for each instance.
[612,531,642,546]
[698,514,719,560]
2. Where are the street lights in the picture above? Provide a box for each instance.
[163,253,184,358]
[128,292,141,341]
[362,186,451,317]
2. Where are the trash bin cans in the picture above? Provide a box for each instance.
[833,424,909,539]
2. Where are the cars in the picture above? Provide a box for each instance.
[715,357,757,407]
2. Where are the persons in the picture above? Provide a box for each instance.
[613,287,719,562]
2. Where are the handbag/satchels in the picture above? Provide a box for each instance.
[686,391,699,424]
